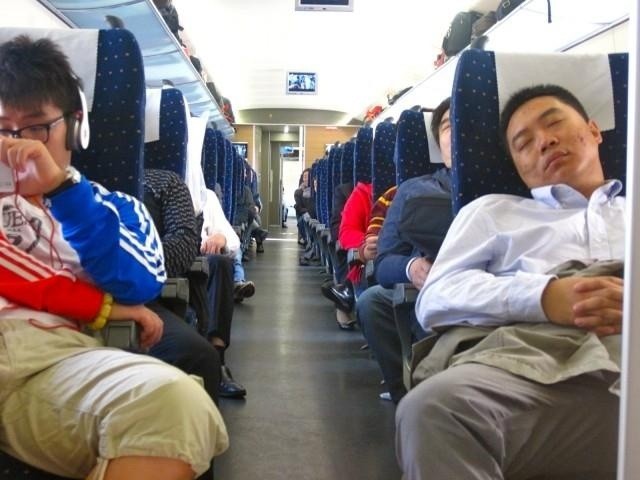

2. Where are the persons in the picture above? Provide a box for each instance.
[395,83,626,480]
[143,167,222,480]
[294,168,317,265]
[372,97,454,340]
[321,182,408,404]
[232,185,268,304]
[294,75,306,89]
[201,188,247,399]
[0,33,229,480]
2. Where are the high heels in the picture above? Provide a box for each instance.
[336,314,354,330]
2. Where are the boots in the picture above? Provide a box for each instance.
[323,282,353,310]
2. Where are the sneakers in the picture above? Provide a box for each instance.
[233,281,255,302]
[257,230,268,252]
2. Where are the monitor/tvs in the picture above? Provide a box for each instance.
[232,141,248,161]
[281,149,299,161]
[286,70,319,96]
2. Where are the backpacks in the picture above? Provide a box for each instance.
[442,0,522,57]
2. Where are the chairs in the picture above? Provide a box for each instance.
[295,49,626,394]
[0,21,260,352]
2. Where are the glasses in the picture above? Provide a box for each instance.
[0,116,64,144]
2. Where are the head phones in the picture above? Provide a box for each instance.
[66,73,91,154]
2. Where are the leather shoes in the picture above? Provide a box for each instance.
[219,366,246,397]
